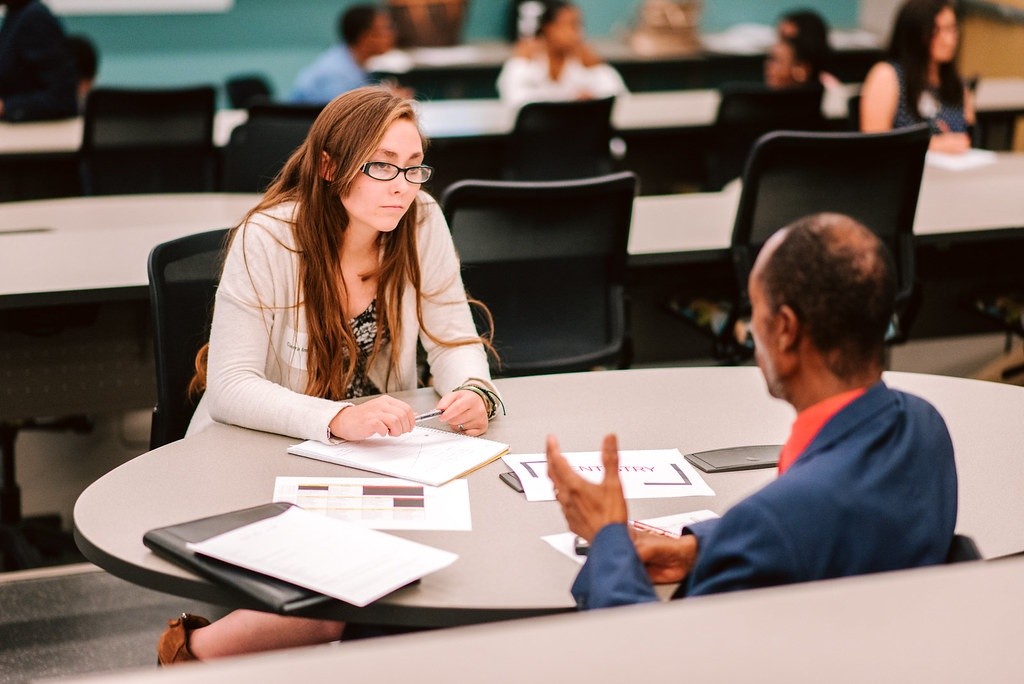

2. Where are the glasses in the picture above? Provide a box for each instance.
[358,162,435,184]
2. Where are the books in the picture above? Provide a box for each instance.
[143,500,421,611]
[287,423,511,488]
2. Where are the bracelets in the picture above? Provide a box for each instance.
[451,386,497,421]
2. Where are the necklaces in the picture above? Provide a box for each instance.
[361,250,372,284]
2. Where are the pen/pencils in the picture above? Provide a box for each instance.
[416,409,444,422]
[0,228,58,234]
[628,520,680,539]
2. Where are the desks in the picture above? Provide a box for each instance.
[0,76,1023,156]
[73,363,1024,626]
[0,154,1023,308]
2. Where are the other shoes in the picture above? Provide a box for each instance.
[157,614,209,666]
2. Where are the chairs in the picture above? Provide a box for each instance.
[75,83,971,450]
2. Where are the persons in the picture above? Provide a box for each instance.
[540,208,957,616]
[287,0,472,112]
[621,1,708,59]
[858,0,973,151]
[158,81,508,669]
[228,74,277,114]
[496,0,632,122]
[696,7,859,190]
[0,0,102,199]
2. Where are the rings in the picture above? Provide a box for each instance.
[554,490,559,496]
[459,425,465,432]
